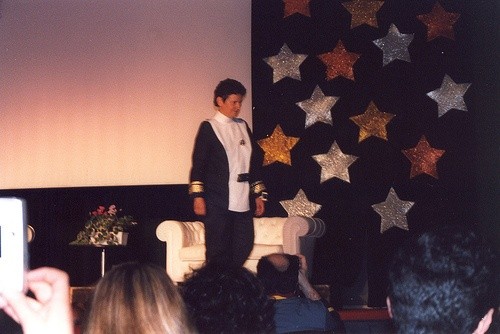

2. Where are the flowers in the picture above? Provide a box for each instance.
[73,205,138,245]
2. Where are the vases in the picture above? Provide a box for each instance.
[90,231,129,245]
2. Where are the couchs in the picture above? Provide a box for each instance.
[156,216,326,286]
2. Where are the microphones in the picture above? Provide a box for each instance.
[240,140,245,144]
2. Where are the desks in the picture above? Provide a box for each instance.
[70,244,129,279]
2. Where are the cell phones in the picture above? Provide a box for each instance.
[0,197,27,294]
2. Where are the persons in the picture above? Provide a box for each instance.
[188,78,269,268]
[0,253,345,333]
[382,225,497,334]
